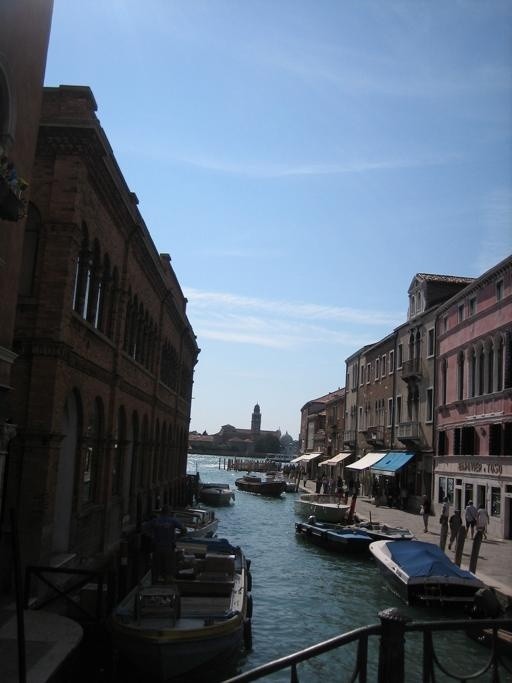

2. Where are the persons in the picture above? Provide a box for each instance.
[474,503,490,540]
[288,461,404,511]
[464,499,477,538]
[440,496,452,518]
[142,504,189,584]
[416,493,429,533]
[448,508,462,550]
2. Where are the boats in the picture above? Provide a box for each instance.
[268,454,297,461]
[235,475,296,496]
[113,509,252,681]
[295,494,512,651]
[199,484,235,507]
